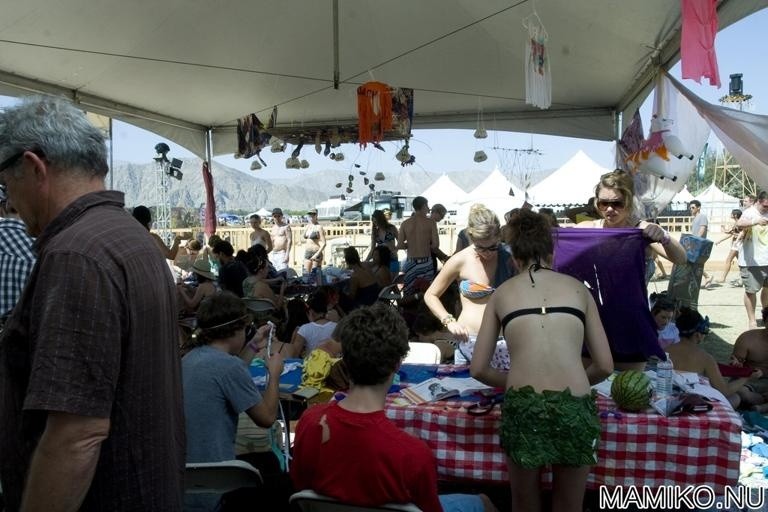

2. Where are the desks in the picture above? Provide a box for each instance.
[248,354,743,512]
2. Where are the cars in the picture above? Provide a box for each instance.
[219,209,311,227]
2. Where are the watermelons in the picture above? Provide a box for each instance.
[611,369,654,412]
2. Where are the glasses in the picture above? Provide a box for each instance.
[759,202,768,208]
[472,244,499,251]
[597,200,626,213]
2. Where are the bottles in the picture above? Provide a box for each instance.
[303,264,334,287]
[656,353,673,401]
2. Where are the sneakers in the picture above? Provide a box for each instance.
[703,275,713,288]
[657,274,668,280]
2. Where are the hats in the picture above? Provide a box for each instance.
[272,208,283,215]
[188,258,218,282]
[307,208,318,214]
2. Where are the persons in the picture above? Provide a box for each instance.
[0,95,187,510]
[173,171,765,511]
[129,204,184,259]
[0,181,42,337]
